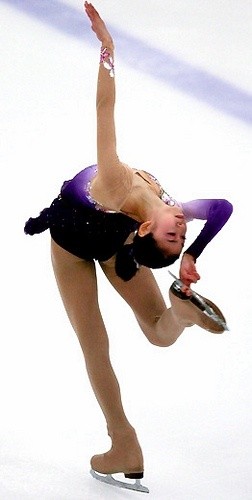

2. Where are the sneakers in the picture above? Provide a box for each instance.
[166,269,228,335]
[89,426,150,493]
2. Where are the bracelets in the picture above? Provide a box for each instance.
[98,47,115,69]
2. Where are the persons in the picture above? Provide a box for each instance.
[22,1,233,493]
